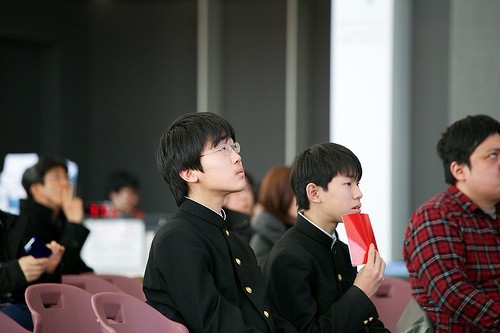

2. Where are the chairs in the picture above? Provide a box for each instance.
[0,274,413,333]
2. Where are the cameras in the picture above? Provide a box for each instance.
[23,236,52,259]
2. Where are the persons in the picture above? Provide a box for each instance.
[221,172,256,245]
[142,112,298,333]
[262,143,392,333]
[402,114,500,333]
[89,170,145,219]
[19,156,94,274]
[248,166,298,274]
[0,209,65,333]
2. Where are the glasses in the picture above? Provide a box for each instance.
[200,142,240,157]
